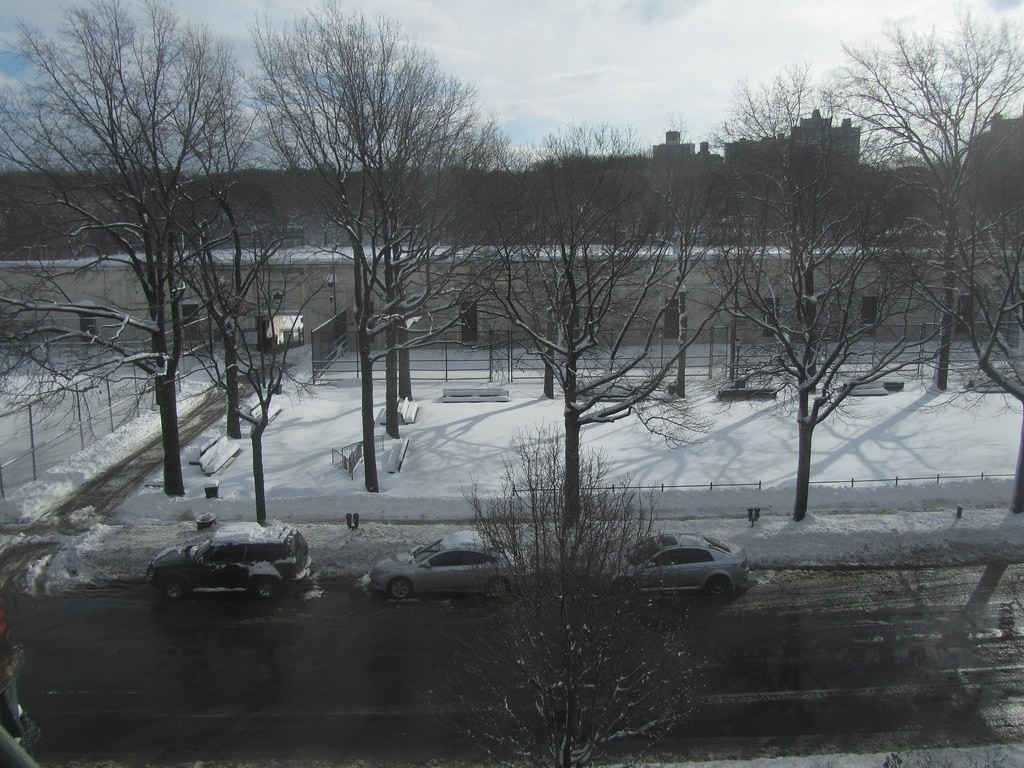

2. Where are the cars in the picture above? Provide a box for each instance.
[369,532,524,603]
[602,532,750,600]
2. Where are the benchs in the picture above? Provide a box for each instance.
[975,380,1024,392]
[594,386,648,400]
[249,398,279,426]
[401,397,418,425]
[841,380,905,393]
[388,439,409,473]
[715,387,776,399]
[194,437,235,477]
[380,396,400,425]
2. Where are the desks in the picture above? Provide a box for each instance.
[442,381,510,397]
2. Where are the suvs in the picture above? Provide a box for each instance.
[145,524,311,601]
